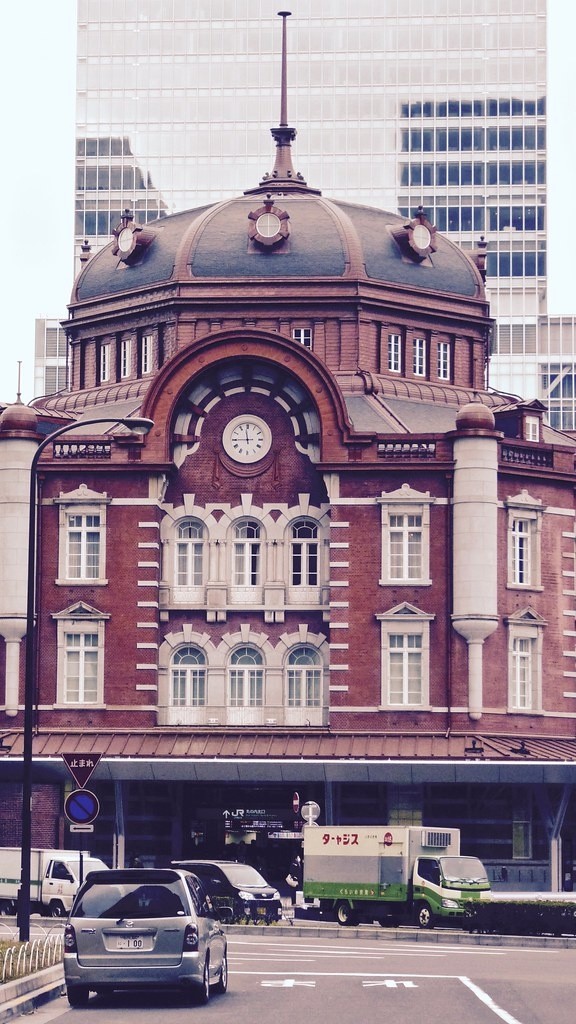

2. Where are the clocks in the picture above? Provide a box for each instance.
[223,414,272,463]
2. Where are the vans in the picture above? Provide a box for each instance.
[170,859,285,925]
[64,867,232,1007]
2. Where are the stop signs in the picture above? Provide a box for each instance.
[292,793,300,813]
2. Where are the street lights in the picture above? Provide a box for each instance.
[17,416,156,946]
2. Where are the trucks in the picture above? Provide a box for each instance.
[0,846,119,918]
[302,824,491,929]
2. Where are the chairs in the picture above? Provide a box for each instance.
[245,899,293,926]
